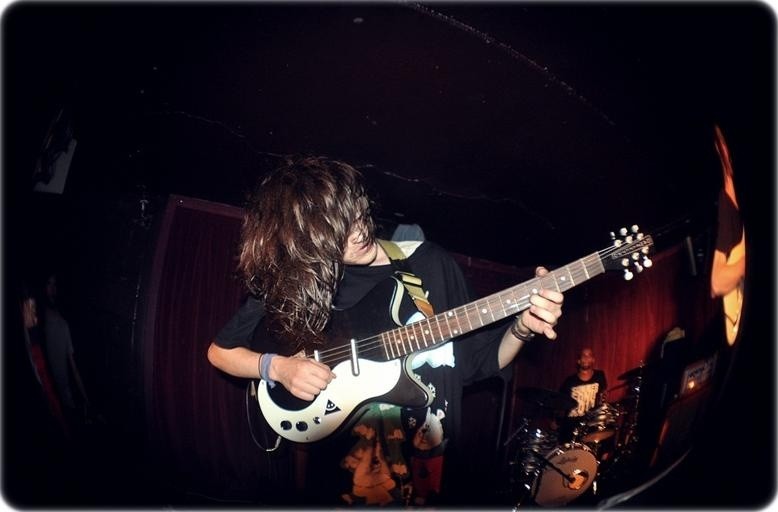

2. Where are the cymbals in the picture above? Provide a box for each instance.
[517,387,563,407]
[617,368,655,379]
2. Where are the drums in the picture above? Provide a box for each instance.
[517,433,598,507]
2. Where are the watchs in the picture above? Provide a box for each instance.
[511,318,533,343]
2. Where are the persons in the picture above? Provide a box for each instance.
[208,157,564,512]
[708,127,751,339]
[40,277,93,414]
[558,349,607,417]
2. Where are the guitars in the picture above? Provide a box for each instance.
[580,406,619,443]
[257,225,655,444]
[721,232,745,342]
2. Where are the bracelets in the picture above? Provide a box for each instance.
[259,353,277,381]
